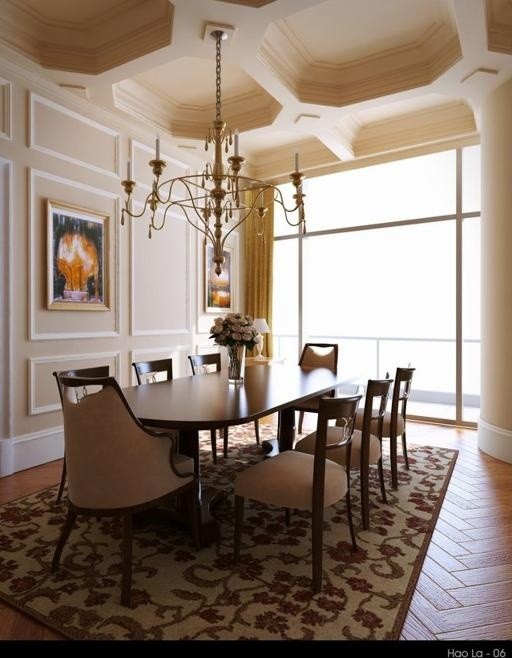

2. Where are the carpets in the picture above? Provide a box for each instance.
[0,421,460,642]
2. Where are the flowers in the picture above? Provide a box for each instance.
[208,312,261,381]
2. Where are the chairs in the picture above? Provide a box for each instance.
[49,376,203,605]
[133,357,174,385]
[298,342,344,436]
[55,366,112,504]
[336,367,416,489]
[294,372,395,533]
[232,387,361,594]
[189,353,229,463]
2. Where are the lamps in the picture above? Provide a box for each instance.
[252,318,272,362]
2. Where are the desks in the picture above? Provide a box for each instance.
[119,367,344,552]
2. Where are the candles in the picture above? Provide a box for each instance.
[231,128,245,158]
[293,152,304,175]
[124,161,135,181]
[204,193,209,210]
[151,180,158,201]
[259,188,265,208]
[155,134,163,164]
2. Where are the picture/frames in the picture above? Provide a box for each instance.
[203,240,239,315]
[44,197,113,313]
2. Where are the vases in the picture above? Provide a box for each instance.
[227,343,249,387]
